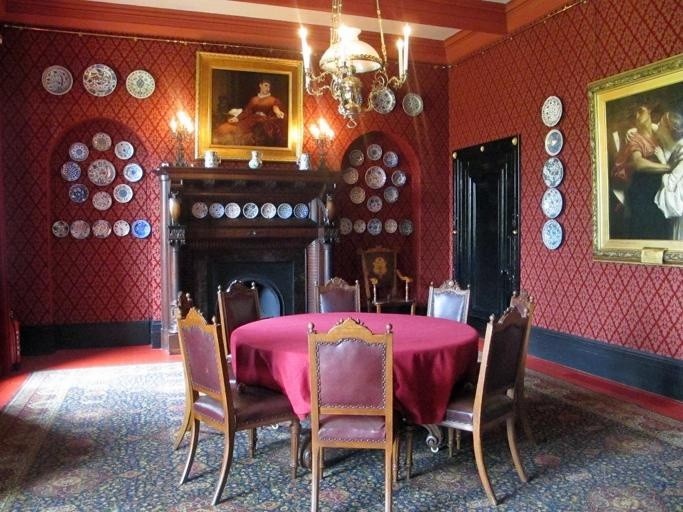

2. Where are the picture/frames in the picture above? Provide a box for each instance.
[195,51,304,162]
[587,53,682,267]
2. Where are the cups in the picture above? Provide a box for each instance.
[204,150,222,169]
[296,153,311,171]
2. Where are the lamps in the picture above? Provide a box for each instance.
[301,0,410,129]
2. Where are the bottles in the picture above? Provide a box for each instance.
[248,150,264,170]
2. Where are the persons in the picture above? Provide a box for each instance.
[228,79,285,146]
[608,103,683,238]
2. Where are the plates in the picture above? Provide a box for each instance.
[539,94,566,252]
[190,200,310,220]
[340,87,426,237]
[40,63,158,241]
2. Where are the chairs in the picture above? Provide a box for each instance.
[217,279,261,381]
[456,289,536,450]
[357,240,418,315]
[176,306,302,506]
[426,279,471,324]
[314,276,361,312]
[403,305,529,507]
[170,291,236,450]
[306,317,400,512]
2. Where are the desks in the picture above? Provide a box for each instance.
[230,311,479,469]
